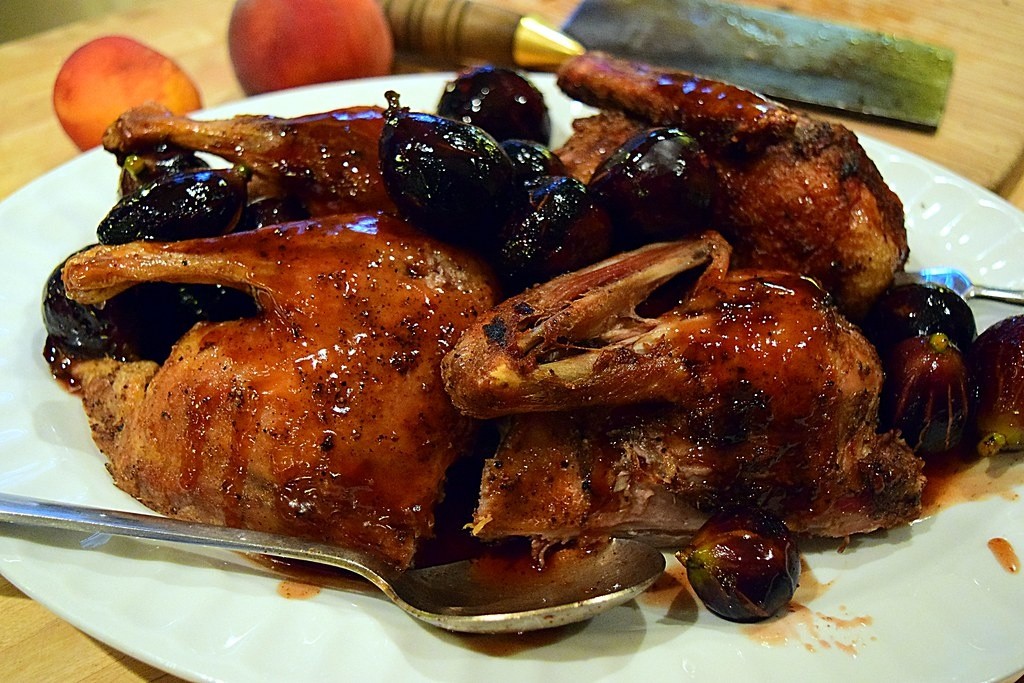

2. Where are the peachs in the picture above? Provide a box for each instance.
[54,1,392,148]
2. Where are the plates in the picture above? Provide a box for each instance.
[0,73,1023,682]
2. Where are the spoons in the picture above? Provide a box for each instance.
[0,485,667,633]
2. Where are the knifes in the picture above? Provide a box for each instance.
[373,1,959,134]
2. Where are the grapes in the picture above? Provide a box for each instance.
[678,514,800,622]
[880,274,1024,461]
[35,151,287,354]
[380,56,717,287]
